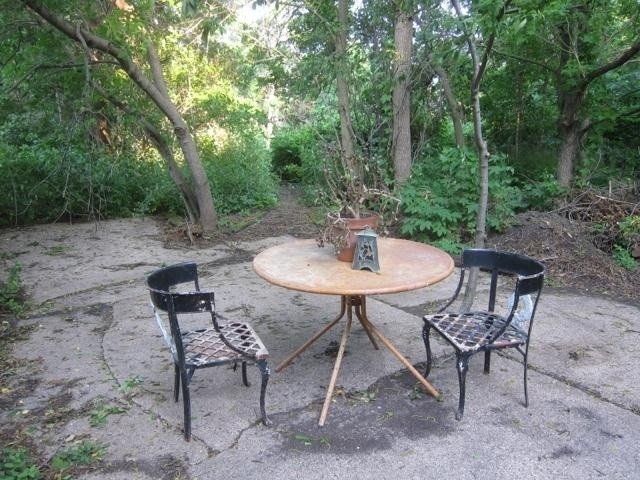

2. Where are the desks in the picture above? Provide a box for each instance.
[253,238,455,426]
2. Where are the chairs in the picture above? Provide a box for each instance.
[145,260,271,441]
[423,247,546,421]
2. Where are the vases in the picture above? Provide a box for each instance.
[326,210,380,263]
